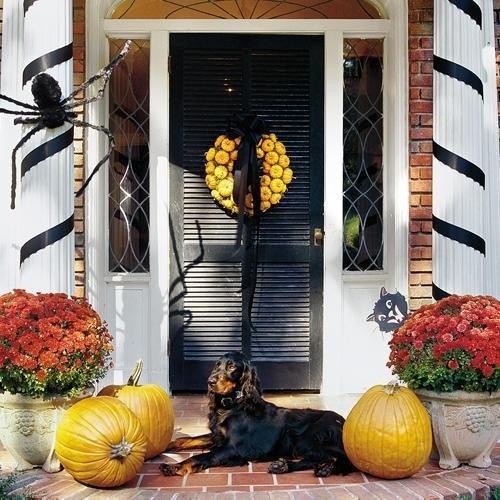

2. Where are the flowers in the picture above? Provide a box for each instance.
[0,288,116,402]
[384,294,500,395]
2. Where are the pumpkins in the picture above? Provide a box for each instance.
[341,381,440,479]
[54,360,173,490]
[204,134,295,215]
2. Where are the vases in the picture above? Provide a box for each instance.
[0,381,96,475]
[411,386,500,473]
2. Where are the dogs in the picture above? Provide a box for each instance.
[158,351,358,479]
